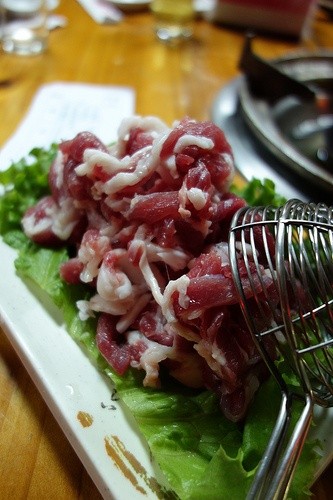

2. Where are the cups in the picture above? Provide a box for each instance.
[151,0,195,42]
[1,1,47,56]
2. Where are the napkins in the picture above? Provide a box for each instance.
[0,81,135,179]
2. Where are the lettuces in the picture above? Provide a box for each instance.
[0,141,333,500]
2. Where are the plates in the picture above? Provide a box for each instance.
[0,234,168,500]
